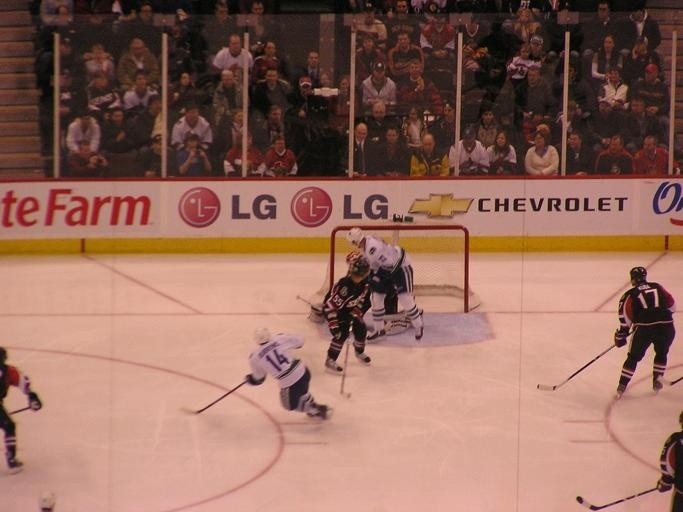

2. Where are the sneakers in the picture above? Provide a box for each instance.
[617,384,625,392]
[325,356,342,371]
[8,459,23,469]
[355,350,371,363]
[319,405,327,420]
[414,321,424,340]
[367,329,385,340]
[653,381,662,390]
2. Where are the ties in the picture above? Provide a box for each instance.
[357,142,363,174]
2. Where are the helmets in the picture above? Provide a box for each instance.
[253,327,268,345]
[462,127,474,141]
[629,267,647,286]
[349,255,370,277]
[346,227,365,247]
[183,130,198,145]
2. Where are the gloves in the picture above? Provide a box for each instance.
[613,329,627,347]
[656,480,672,493]
[28,392,42,410]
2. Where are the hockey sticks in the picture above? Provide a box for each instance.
[655,375,683,392]
[576,488,677,511]
[535,331,631,391]
[340,328,352,399]
[180,378,248,416]
[7,407,36,416]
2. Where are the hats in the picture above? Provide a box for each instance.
[299,77,311,87]
[372,60,383,70]
[644,64,657,75]
[38,491,55,509]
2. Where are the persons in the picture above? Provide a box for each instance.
[37,2,683,176]
[657,410,683,512]
[246,327,326,420]
[0,348,42,475]
[323,254,371,373]
[39,491,56,512]
[614,267,676,400]
[347,228,424,339]
